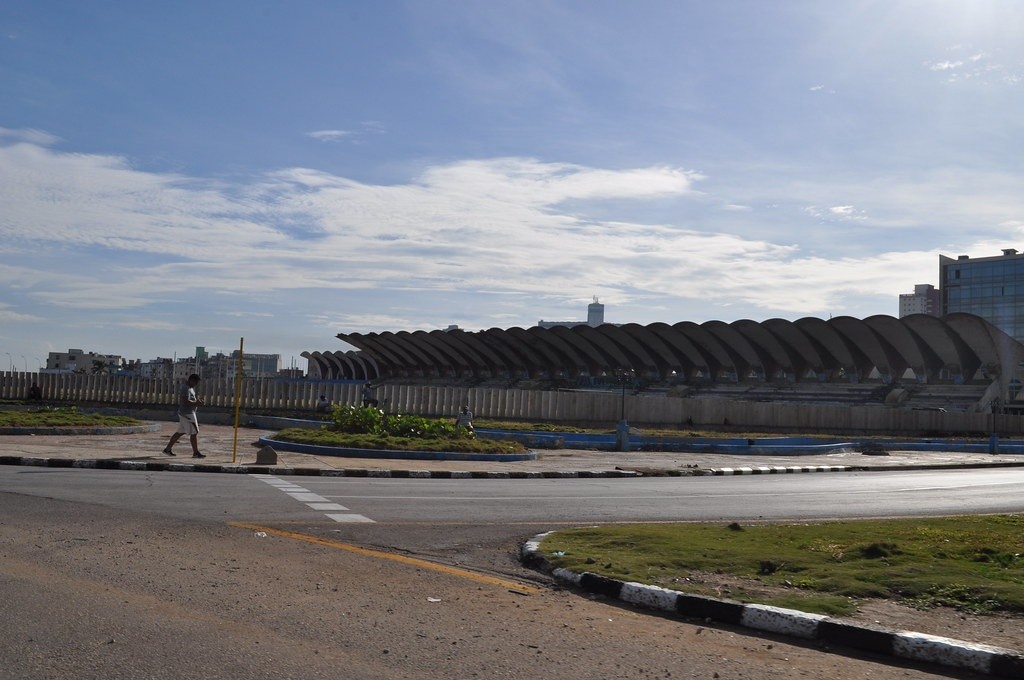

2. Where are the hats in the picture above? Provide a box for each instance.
[365,383,372,387]
[463,406,469,411]
[320,395,326,398]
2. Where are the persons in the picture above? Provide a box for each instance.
[27,383,41,401]
[316,395,331,413]
[454,406,475,432]
[162,374,207,459]
[360,383,384,408]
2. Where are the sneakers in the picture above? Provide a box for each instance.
[162,448,176,457]
[192,451,206,458]
